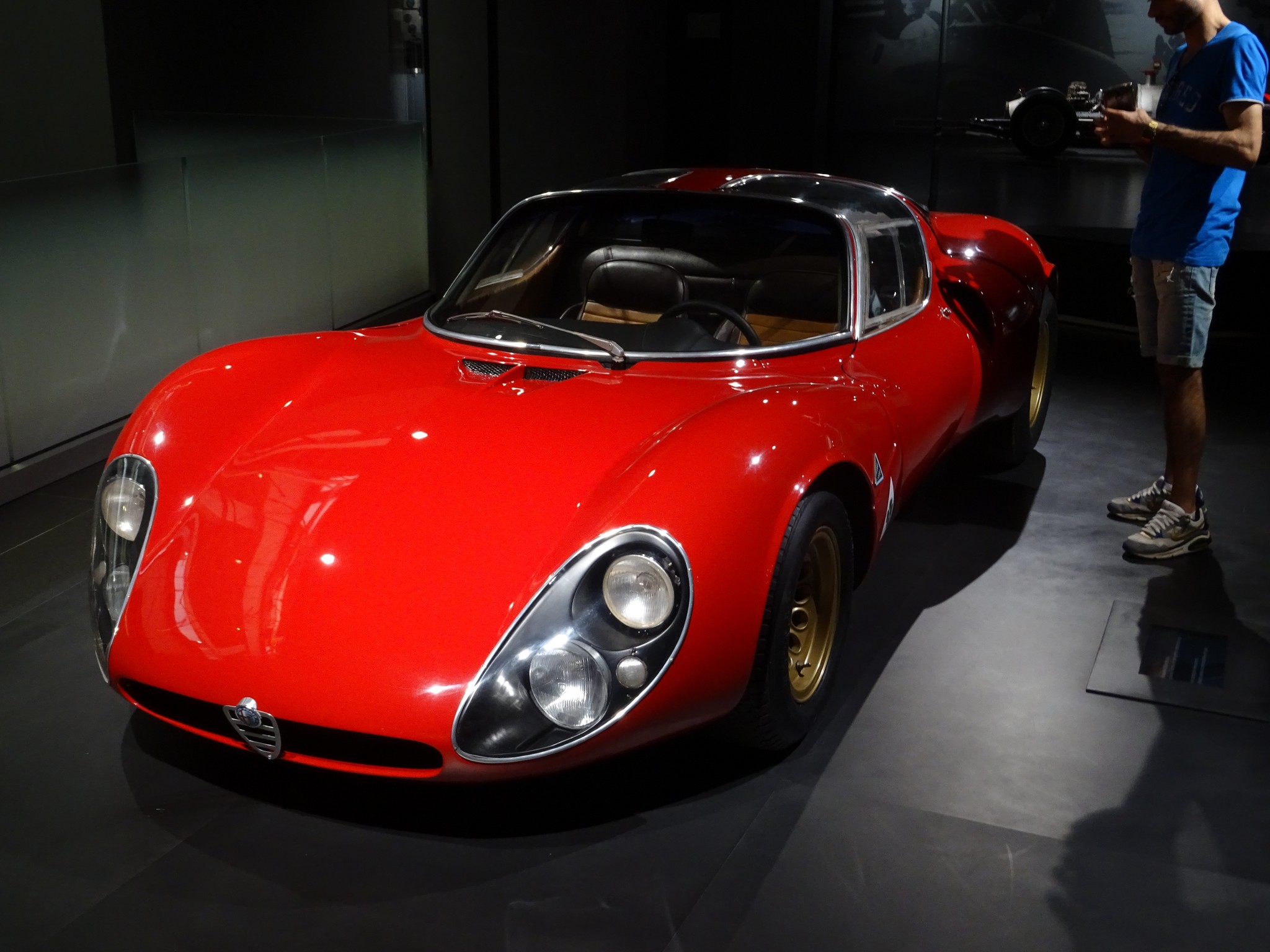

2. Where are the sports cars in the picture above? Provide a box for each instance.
[82,162,1064,791]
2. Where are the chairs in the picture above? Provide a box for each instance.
[712,253,881,349]
[556,241,730,344]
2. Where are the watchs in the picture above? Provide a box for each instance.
[1142,121,1159,142]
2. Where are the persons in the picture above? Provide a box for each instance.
[1088,0,1270,561]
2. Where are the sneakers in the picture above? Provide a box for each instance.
[1122,499,1211,558]
[1106,474,1208,522]
